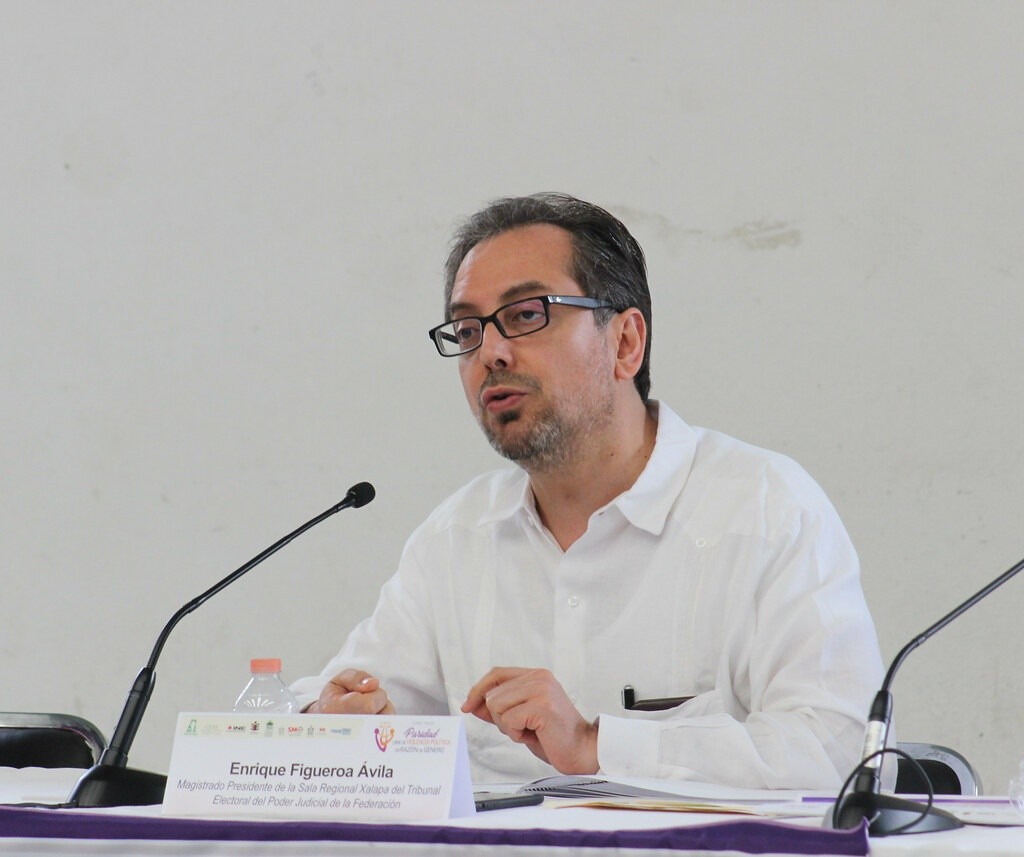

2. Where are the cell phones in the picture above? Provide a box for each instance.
[473,792,543,813]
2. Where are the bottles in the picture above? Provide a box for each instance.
[233,657,299,714]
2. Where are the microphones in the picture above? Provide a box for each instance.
[67,481,377,809]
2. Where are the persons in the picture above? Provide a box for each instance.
[269,191,898,796]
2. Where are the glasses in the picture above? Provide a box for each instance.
[427,294,624,357]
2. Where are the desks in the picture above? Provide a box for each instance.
[0,783,1024,857]
